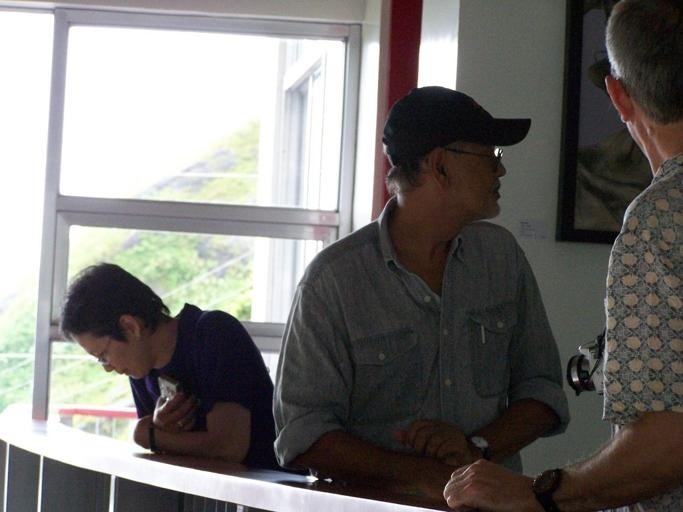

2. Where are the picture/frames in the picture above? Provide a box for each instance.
[555,1,653,243]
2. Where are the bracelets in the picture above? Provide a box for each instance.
[149,426,155,451]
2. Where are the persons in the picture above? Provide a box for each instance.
[271,86,570,507]
[442,0,683,511]
[58,263,310,476]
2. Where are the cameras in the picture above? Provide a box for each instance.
[567,330,606,394]
[157,373,182,402]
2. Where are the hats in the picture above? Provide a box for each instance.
[381,87,531,168]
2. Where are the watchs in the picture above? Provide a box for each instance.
[531,469,561,512]
[466,435,491,460]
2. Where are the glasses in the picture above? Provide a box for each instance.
[94,338,112,363]
[445,148,503,172]
